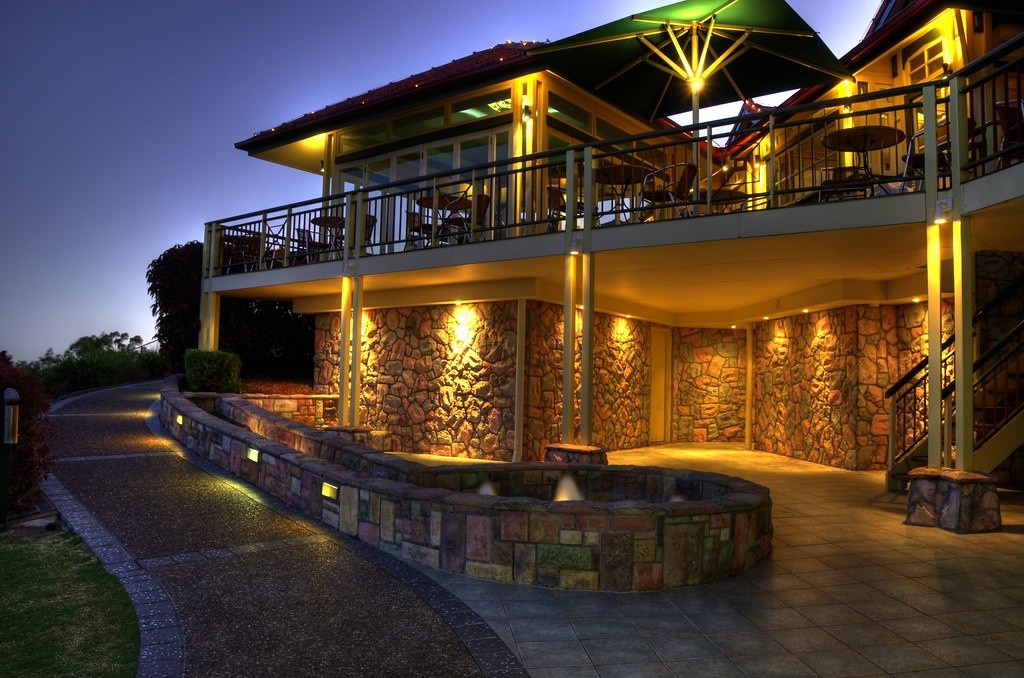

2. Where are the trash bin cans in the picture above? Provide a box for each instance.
[3,389,20,444]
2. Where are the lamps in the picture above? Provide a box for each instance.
[523,104,531,120]
[321,160,324,173]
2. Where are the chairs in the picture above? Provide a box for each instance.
[221,97,1024,273]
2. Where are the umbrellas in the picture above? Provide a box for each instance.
[523,0,856,216]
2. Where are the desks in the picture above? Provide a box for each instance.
[592,166,654,226]
[221,233,261,269]
[820,124,906,201]
[310,216,345,261]
[688,187,749,206]
[416,196,472,248]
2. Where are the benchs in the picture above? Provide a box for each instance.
[900,467,1001,536]
[324,426,373,447]
[544,444,608,466]
